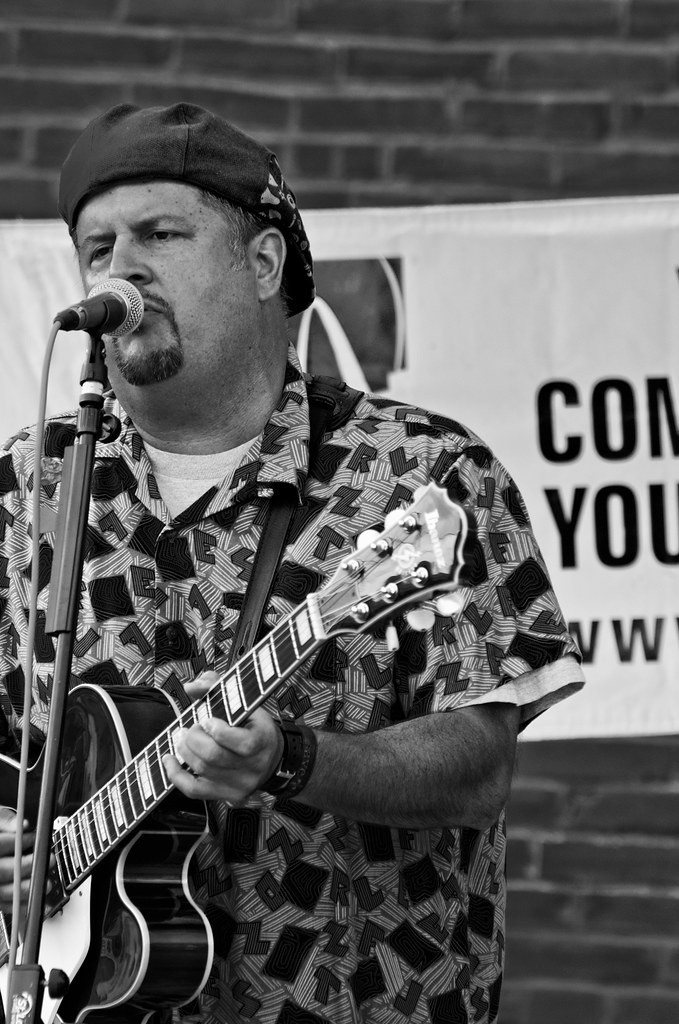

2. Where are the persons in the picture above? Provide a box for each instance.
[0,105,589,1024]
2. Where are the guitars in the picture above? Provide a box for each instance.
[0,476,492,1024]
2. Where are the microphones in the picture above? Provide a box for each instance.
[53,277,145,337]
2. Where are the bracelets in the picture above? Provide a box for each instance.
[280,726,317,801]
[260,711,299,796]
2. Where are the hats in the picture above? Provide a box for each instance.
[56,104,317,319]
